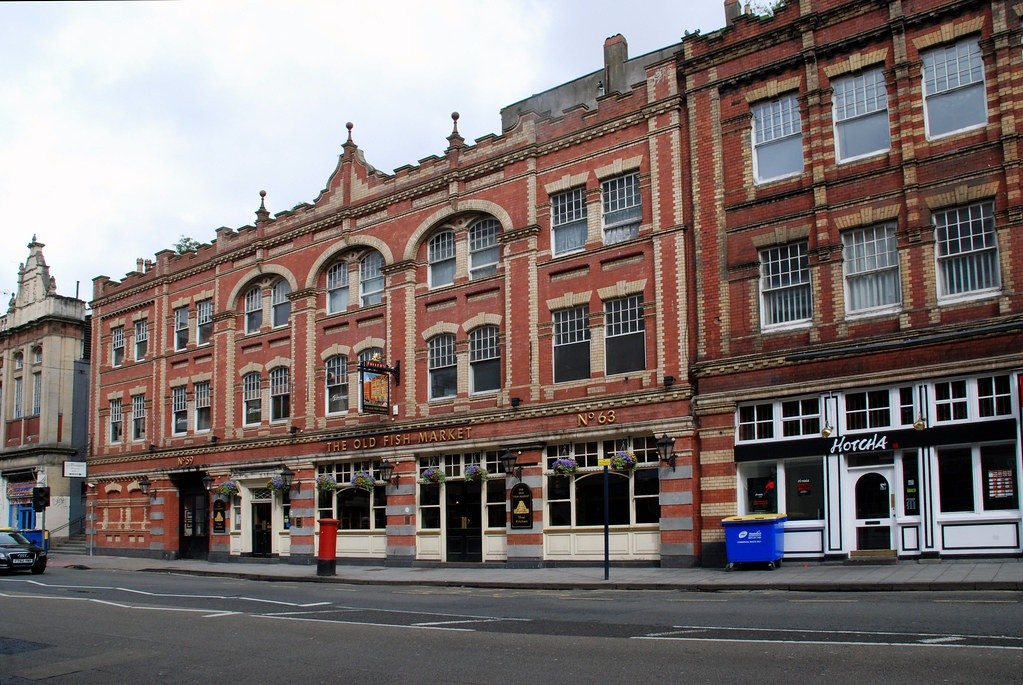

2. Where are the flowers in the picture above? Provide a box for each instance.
[316,474,335,491]
[464,466,488,483]
[217,480,238,495]
[610,451,637,472]
[553,459,576,478]
[352,471,375,493]
[267,475,283,492]
[422,469,446,488]
[173,235,200,252]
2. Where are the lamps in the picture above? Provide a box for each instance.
[210,436,220,442]
[139,475,157,501]
[203,472,217,495]
[280,466,301,494]
[913,412,925,430]
[664,376,676,388]
[378,459,399,490]
[289,426,300,434]
[822,420,834,438]
[148,444,158,450]
[512,397,523,407]
[501,447,522,482]
[655,433,676,472]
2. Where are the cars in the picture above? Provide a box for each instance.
[0,530,47,575]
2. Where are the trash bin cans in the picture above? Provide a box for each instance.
[21,529,50,552]
[722,513,788,573]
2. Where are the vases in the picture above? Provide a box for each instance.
[557,468,565,474]
[428,476,439,482]
[612,464,635,470]
[473,472,480,480]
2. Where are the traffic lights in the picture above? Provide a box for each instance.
[32,487,46,512]
[38,487,50,507]
